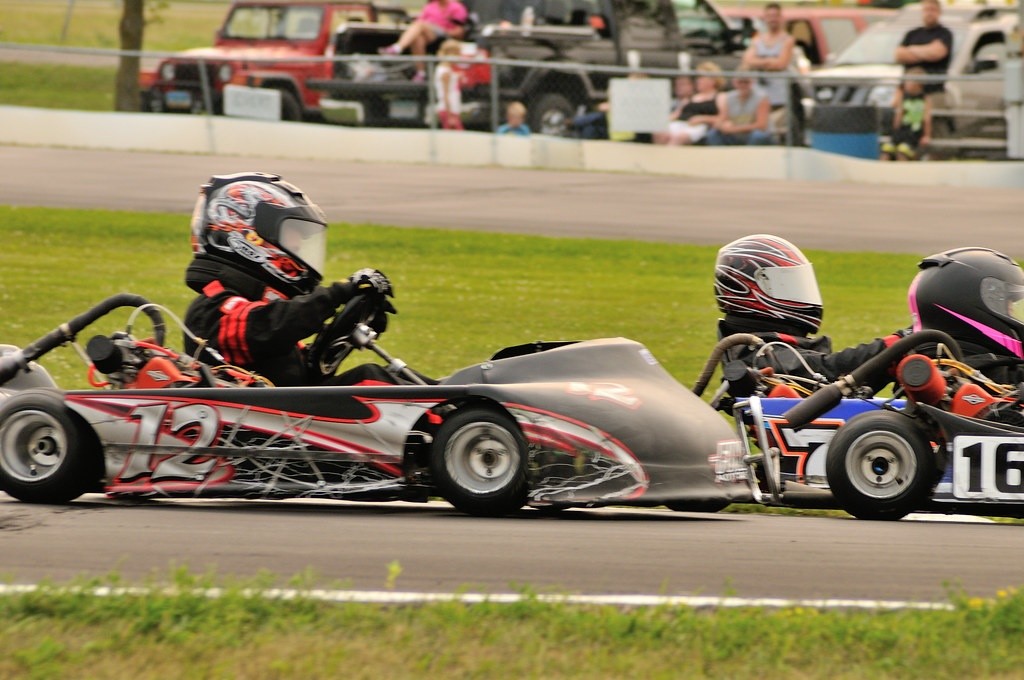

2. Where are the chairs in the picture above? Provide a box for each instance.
[716,318,773,397]
[295,17,318,38]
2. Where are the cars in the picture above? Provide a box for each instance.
[805,1,1022,137]
[677,8,895,64]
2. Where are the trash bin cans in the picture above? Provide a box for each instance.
[812,105,879,160]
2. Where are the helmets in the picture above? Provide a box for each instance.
[185,170,327,297]
[711,232,824,337]
[909,246,1024,366]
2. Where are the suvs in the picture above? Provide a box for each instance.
[140,1,413,125]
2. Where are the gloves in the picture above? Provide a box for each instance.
[350,267,391,300]
[366,313,388,340]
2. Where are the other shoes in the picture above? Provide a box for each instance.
[378,44,398,56]
[412,72,428,85]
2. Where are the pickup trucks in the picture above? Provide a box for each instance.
[305,0,754,137]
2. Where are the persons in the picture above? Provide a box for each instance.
[497,102,530,134]
[566,63,781,144]
[909,247,1024,389]
[970,8,1004,73]
[716,234,913,396]
[740,3,804,145]
[378,0,468,80]
[433,39,463,129]
[880,67,932,161]
[898,0,956,134]
[185,171,438,385]
[788,47,810,147]
